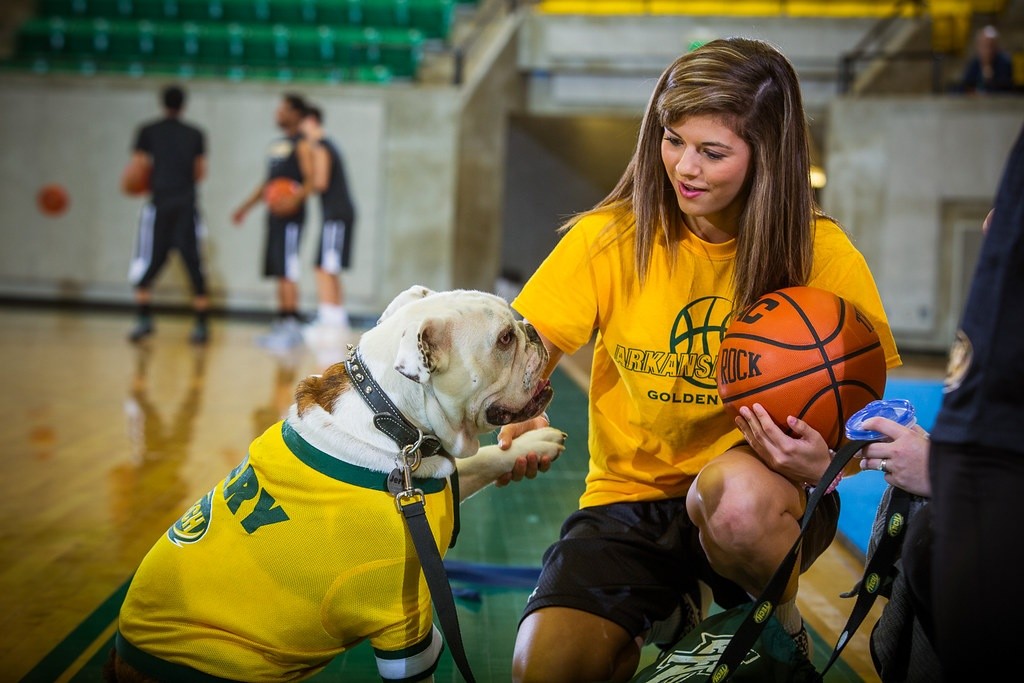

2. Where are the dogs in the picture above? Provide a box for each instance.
[114,285,569,683]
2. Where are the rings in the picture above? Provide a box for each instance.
[881,458,889,472]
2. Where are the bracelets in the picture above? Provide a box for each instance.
[800,449,847,496]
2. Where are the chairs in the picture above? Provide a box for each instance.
[0,0,457,84]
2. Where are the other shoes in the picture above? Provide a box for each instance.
[302,302,354,346]
[129,308,157,342]
[190,315,211,346]
[656,580,713,662]
[258,310,304,352]
[788,615,815,664]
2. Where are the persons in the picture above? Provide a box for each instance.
[298,106,357,344]
[126,85,210,346]
[860,121,1024,683]
[497,37,902,683]
[231,92,308,348]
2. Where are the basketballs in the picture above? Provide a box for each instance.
[36,185,69,218]
[122,162,153,196]
[716,286,886,449]
[265,180,298,213]
